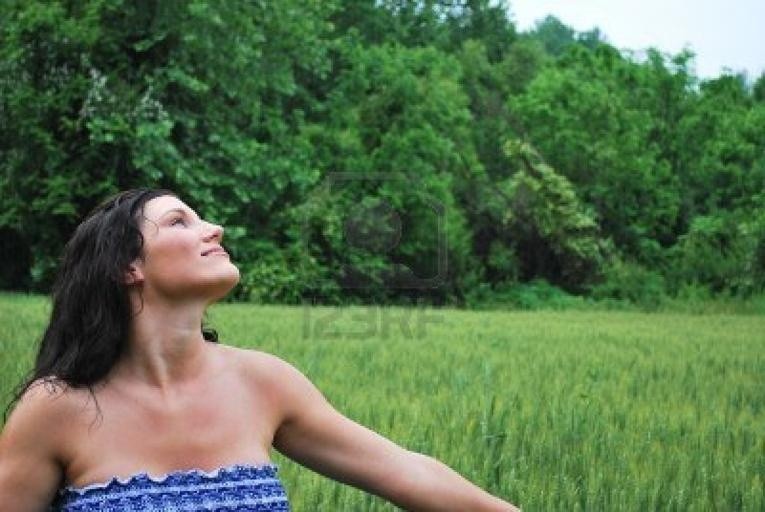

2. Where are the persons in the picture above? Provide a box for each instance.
[1,187,519,512]
